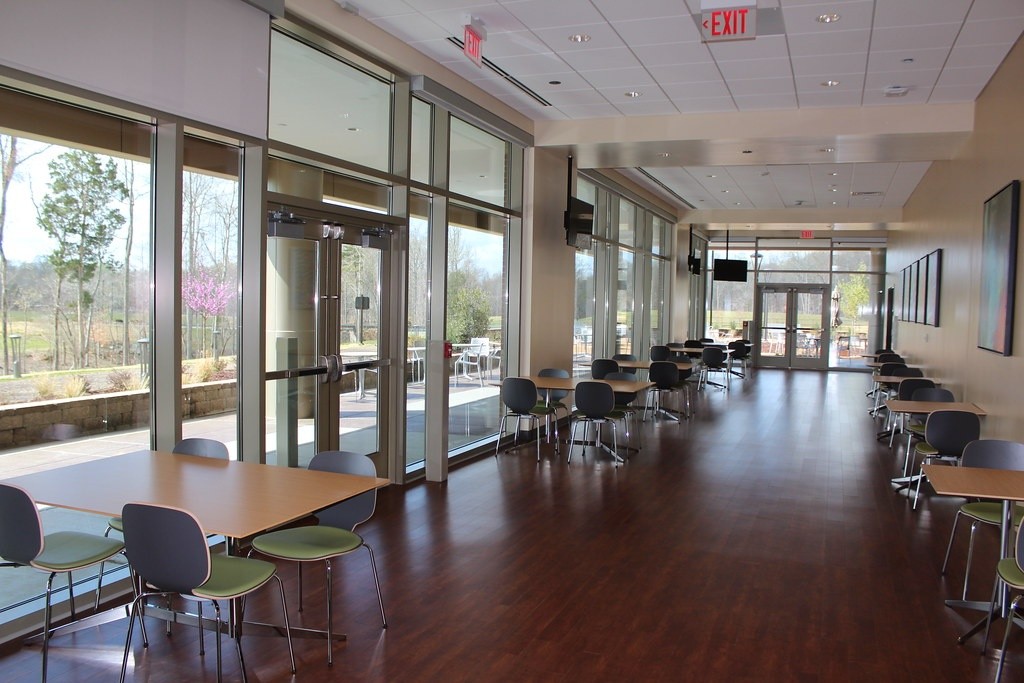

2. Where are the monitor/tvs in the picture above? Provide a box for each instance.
[567,196,594,250]
[692,248,701,276]
[714,259,747,282]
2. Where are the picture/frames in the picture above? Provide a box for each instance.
[898,248,942,327]
[977,180,1020,357]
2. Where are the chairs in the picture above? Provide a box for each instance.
[341,342,377,399]
[889,379,936,449]
[903,387,955,477]
[887,368,924,429]
[871,349,895,390]
[93,437,229,636]
[0,480,150,683]
[940,439,1024,599]
[873,354,901,399]
[413,340,425,383]
[239,450,388,669]
[873,364,908,420]
[980,516,1024,683]
[906,408,981,510]
[119,503,298,683]
[768,330,867,364]
[455,325,753,469]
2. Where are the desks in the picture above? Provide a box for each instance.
[0,450,392,683]
[866,362,923,399]
[873,375,942,443]
[883,399,988,493]
[680,342,755,379]
[860,354,909,359]
[406,346,425,352]
[578,360,698,421]
[491,376,658,463]
[578,334,592,358]
[648,347,735,391]
[341,351,377,400]
[451,344,488,349]
[919,464,1024,646]
